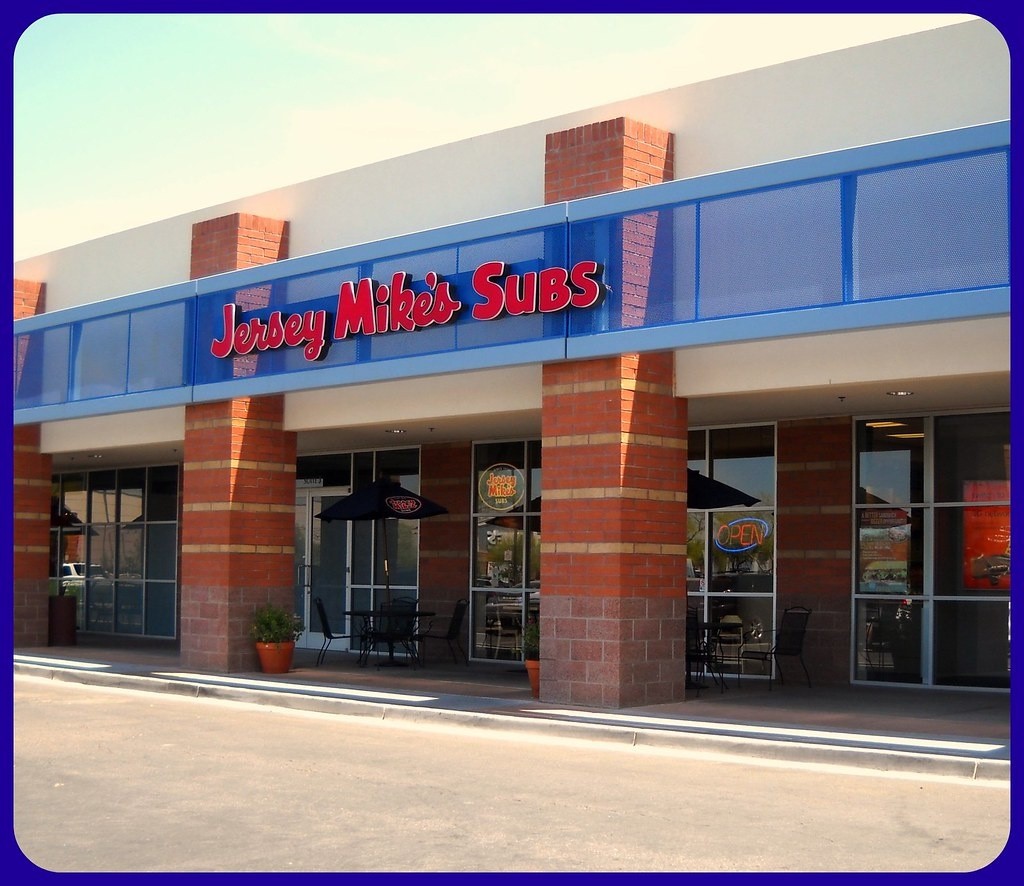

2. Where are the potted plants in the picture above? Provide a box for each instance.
[519,613,539,698]
[250,602,306,674]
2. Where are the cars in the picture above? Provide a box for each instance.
[62,563,107,592]
[687,572,774,643]
[861,580,910,651]
[484,580,540,626]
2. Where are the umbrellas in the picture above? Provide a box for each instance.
[487,494,540,533]
[314,477,455,661]
[685,467,761,512]
[855,487,914,532]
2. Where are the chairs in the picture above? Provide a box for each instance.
[684,601,813,697]
[486,602,519,660]
[313,592,475,671]
[857,614,900,673]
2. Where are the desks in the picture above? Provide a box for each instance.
[341,608,437,668]
